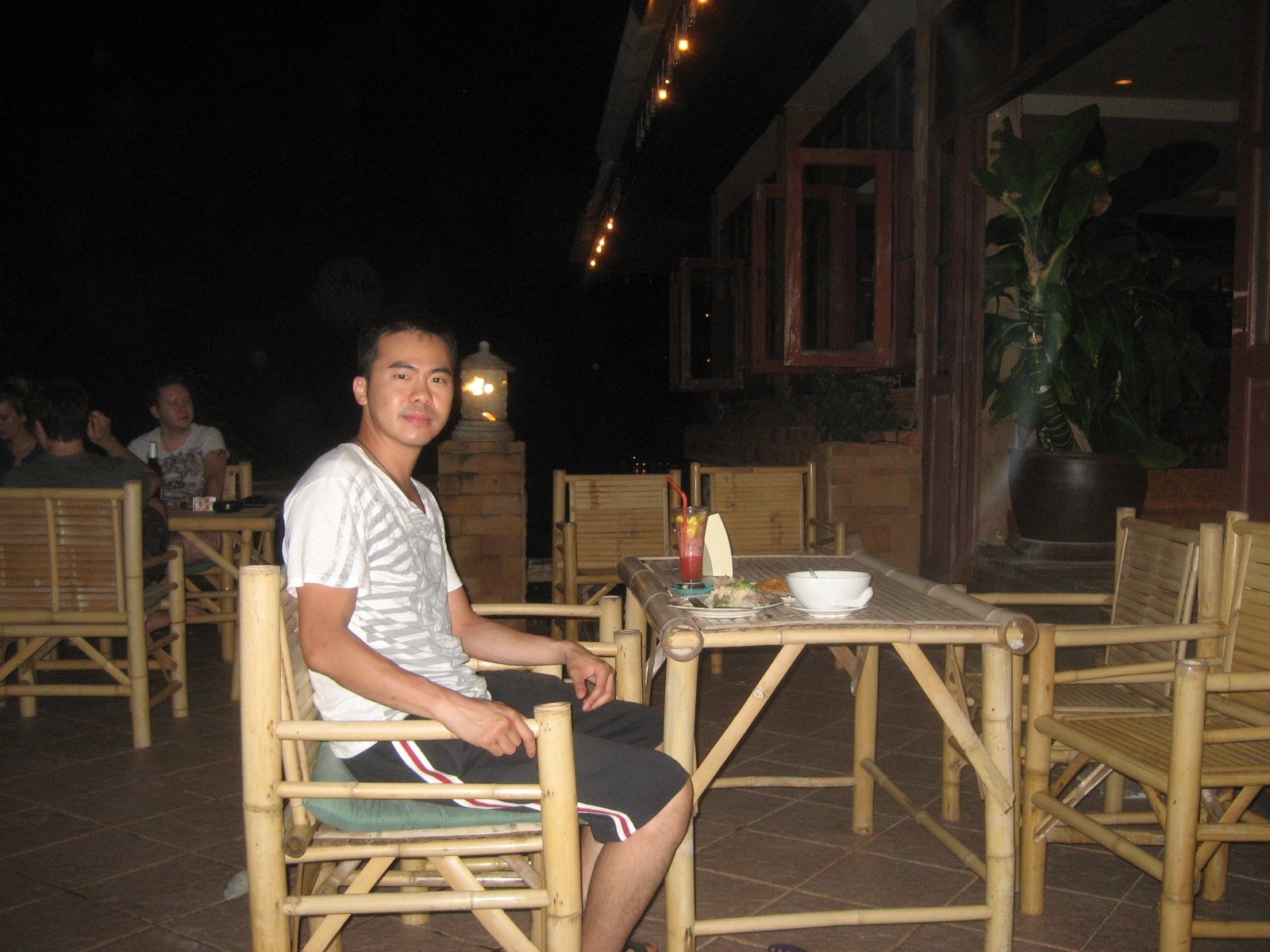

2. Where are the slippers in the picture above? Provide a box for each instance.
[623,940,648,952]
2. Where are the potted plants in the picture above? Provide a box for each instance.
[965,104,1235,543]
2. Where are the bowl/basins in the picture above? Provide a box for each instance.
[212,500,243,513]
[783,570,872,610]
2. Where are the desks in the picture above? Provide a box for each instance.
[166,502,284,702]
[616,547,1039,952]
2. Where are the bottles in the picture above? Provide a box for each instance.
[146,441,164,503]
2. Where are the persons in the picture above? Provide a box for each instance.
[0,373,230,671]
[281,316,694,951]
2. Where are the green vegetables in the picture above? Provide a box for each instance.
[713,581,761,608]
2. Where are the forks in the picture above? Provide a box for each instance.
[688,597,752,609]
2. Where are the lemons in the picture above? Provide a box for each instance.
[676,513,706,538]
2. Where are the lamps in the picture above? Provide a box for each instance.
[451,340,516,421]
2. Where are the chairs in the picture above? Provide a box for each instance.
[551,461,847,674]
[238,565,643,952]
[948,506,1270,952]
[0,460,253,749]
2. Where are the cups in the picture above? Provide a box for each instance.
[672,505,709,581]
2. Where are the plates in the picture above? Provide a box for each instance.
[665,593,785,618]
[790,601,869,617]
[671,582,715,594]
